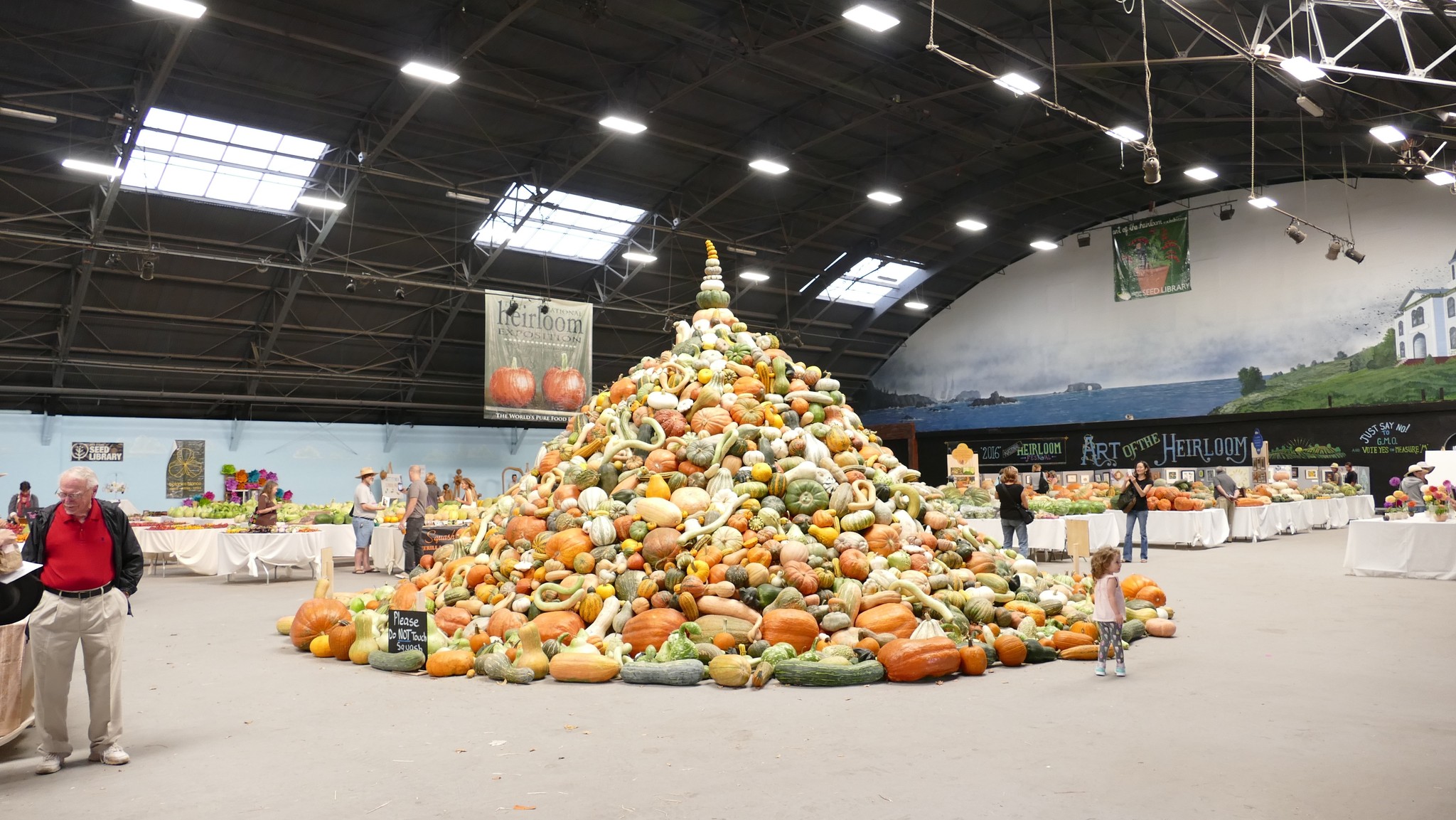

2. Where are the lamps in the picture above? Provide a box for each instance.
[346,279,358,293]
[138,251,155,283]
[775,331,783,341]
[394,284,405,300]
[1295,94,1325,117]
[1075,231,1091,248]
[102,251,124,270]
[1325,235,1344,261]
[1279,0,1326,82]
[1424,170,1456,187]
[1368,124,1407,144]
[662,315,677,334]
[789,333,804,348]
[1284,218,1309,248]
[1219,201,1235,221]
[1141,144,1166,186]
[506,296,518,317]
[540,298,550,315]
[1346,244,1367,267]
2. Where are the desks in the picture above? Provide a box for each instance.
[964,485,1456,581]
[371,524,418,575]
[163,515,196,524]
[195,517,237,525]
[317,521,364,559]
[130,522,238,579]
[217,528,322,584]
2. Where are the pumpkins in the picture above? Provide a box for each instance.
[490,356,536,407]
[589,238,846,409]
[1235,471,1365,507]
[542,352,587,410]
[1144,478,1218,511]
[271,393,1175,688]
[166,497,260,523]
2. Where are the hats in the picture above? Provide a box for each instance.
[1330,462,1338,468]
[355,468,379,478]
[1417,461,1435,474]
[1404,464,1429,477]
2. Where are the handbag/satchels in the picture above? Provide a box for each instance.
[349,504,354,517]
[1116,483,1136,513]
[1039,471,1049,494]
[1018,507,1034,524]
[248,506,259,526]
[461,489,476,508]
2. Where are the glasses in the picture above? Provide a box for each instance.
[54,486,91,500]
[368,474,375,477]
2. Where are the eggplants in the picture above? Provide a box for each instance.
[239,525,273,533]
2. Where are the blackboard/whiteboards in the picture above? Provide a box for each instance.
[387,609,428,670]
[1066,519,1090,557]
[1425,450,1456,487]
[24,507,44,531]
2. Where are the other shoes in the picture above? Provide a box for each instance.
[1227,541,1231,543]
[395,572,409,579]
[1222,541,1227,543]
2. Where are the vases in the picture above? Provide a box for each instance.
[1424,510,1431,517]
[1434,514,1448,522]
[1428,505,1437,521]
[1384,512,1408,520]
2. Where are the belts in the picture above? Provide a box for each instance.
[1216,495,1223,500]
[43,580,115,598]
[355,516,373,521]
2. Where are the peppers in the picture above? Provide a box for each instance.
[4,522,30,543]
[130,521,236,530]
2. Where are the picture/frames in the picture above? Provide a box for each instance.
[983,467,1347,490]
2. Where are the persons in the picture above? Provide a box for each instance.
[508,474,517,489]
[1344,462,1357,487]
[441,483,453,501]
[1214,466,1240,542]
[396,465,429,579]
[1090,546,1127,676]
[995,466,1029,559]
[1327,462,1343,486]
[994,468,1004,486]
[1399,461,1456,514]
[256,481,282,527]
[453,469,462,499]
[351,467,387,574]
[1029,464,1057,494]
[16,465,144,773]
[946,475,956,488]
[454,478,478,509]
[8,481,39,524]
[424,472,444,510]
[1120,460,1154,564]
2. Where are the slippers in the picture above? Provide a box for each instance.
[1120,559,1131,563]
[1141,559,1147,562]
[365,568,380,573]
[352,570,365,574]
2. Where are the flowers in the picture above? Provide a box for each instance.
[180,461,294,509]
[1381,474,1453,514]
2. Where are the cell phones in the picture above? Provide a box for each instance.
[1127,469,1132,477]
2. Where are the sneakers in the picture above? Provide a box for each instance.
[35,754,64,774]
[1114,667,1126,676]
[1095,668,1106,675]
[88,743,129,765]
